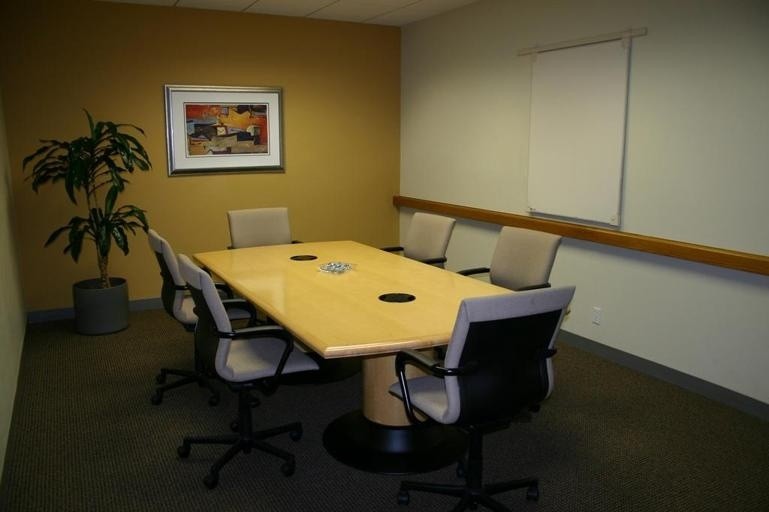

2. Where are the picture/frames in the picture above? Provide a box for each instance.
[164,80,287,177]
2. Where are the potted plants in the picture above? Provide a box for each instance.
[22,100,159,339]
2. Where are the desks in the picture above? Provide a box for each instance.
[193,240,520,476]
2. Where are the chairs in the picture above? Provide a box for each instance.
[226,207,292,250]
[388,285,575,512]
[378,212,456,270]
[177,253,321,489]
[456,226,562,291]
[147,228,319,407]
